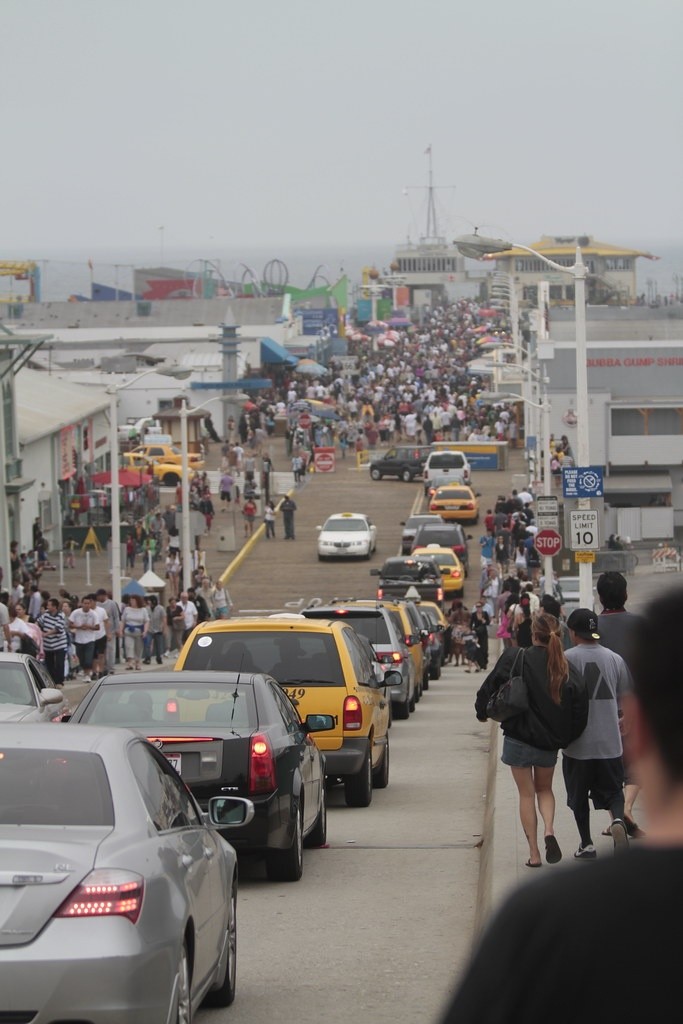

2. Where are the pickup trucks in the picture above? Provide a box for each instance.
[371,555,451,612]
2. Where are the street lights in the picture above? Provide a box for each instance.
[108,365,192,610]
[480,342,554,597]
[180,394,252,591]
[455,234,593,609]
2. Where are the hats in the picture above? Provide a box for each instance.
[567,608,601,641]
[597,571,627,609]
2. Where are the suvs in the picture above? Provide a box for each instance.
[422,450,474,495]
[409,524,473,578]
[166,615,405,806]
[301,603,418,720]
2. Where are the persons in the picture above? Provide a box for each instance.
[439,586,683,1024]
[475,613,589,868]
[563,608,630,859]
[0,294,619,688]
[593,572,647,839]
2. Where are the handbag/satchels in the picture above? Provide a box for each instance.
[485,648,530,722]
[20,633,40,659]
[506,603,517,632]
[171,620,187,632]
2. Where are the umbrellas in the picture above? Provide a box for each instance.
[295,358,327,375]
[482,343,498,350]
[364,321,389,330]
[476,336,501,344]
[313,411,341,419]
[90,467,148,487]
[385,318,411,326]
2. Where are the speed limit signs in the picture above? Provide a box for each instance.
[570,509,600,551]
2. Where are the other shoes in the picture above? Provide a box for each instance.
[53,642,183,690]
[624,815,647,838]
[601,825,612,836]
[445,658,487,673]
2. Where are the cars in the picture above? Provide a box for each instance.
[347,599,451,701]
[118,426,207,488]
[414,543,466,598]
[0,652,336,882]
[400,514,445,555]
[0,720,252,1024]
[370,444,435,482]
[426,474,482,524]
[358,634,394,730]
[315,512,377,561]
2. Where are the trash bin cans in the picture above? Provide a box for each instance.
[215,525,236,552]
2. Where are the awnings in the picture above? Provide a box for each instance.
[259,338,291,363]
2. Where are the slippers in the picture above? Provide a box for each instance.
[525,858,542,867]
[544,835,562,864]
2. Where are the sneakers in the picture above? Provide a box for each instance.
[611,818,630,856]
[573,842,596,861]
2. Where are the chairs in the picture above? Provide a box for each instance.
[206,705,230,722]
[95,703,137,723]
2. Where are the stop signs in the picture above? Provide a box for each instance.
[536,530,564,556]
